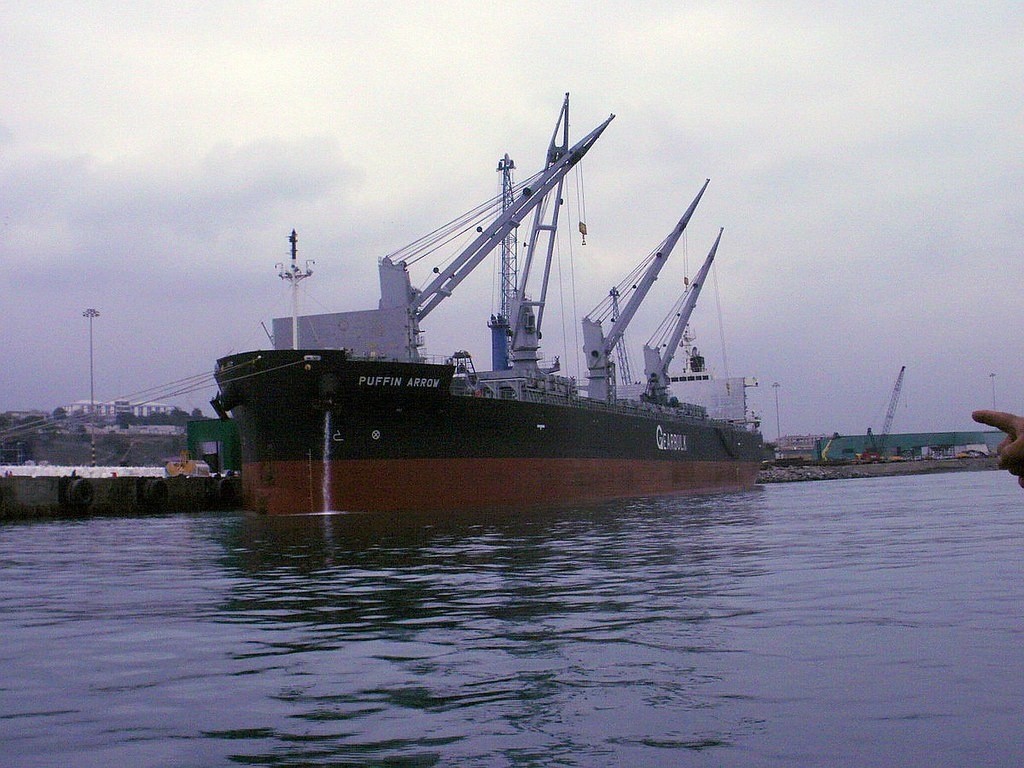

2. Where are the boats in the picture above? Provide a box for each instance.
[212,93,763,518]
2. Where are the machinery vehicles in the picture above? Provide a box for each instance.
[165,449,210,477]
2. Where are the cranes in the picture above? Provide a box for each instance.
[866,364,907,459]
